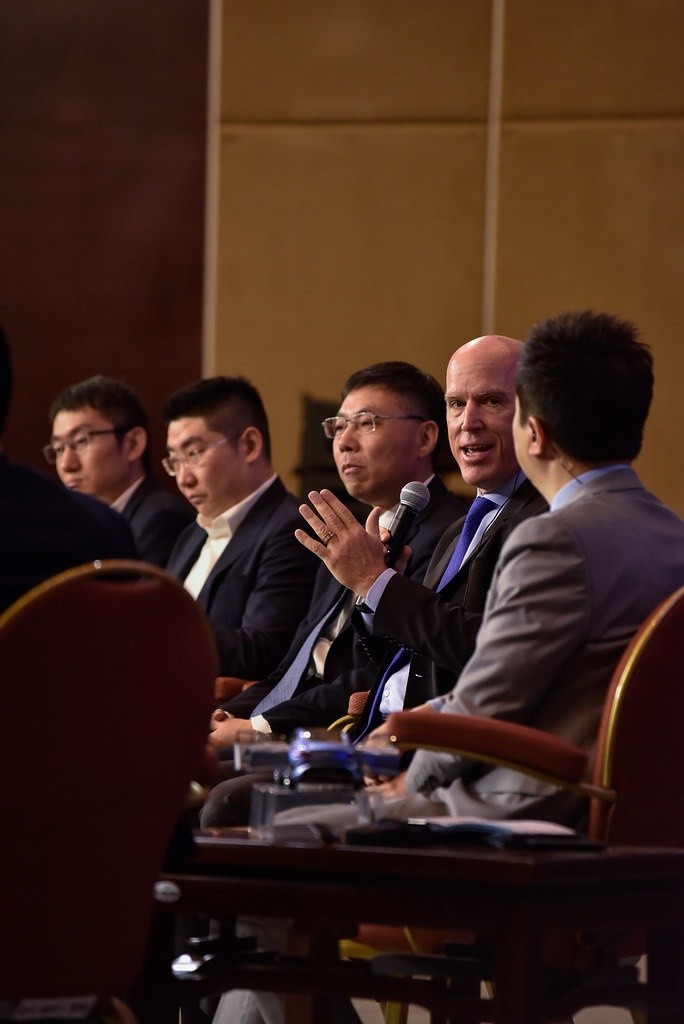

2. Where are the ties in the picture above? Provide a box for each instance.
[248,510,396,715]
[339,496,494,744]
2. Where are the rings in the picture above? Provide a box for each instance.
[322,530,335,544]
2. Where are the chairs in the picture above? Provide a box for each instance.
[362,585,684,1024]
[0,563,223,1024]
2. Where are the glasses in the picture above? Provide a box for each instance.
[42,426,121,465]
[161,433,242,476]
[320,412,423,440]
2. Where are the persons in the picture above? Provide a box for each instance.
[209,310,684,1024]
[0,323,141,617]
[163,376,322,681]
[43,375,195,584]
[200,334,547,833]
[128,362,479,1023]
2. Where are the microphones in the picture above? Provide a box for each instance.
[356,482,429,614]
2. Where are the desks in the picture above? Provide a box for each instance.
[159,834,684,1024]
